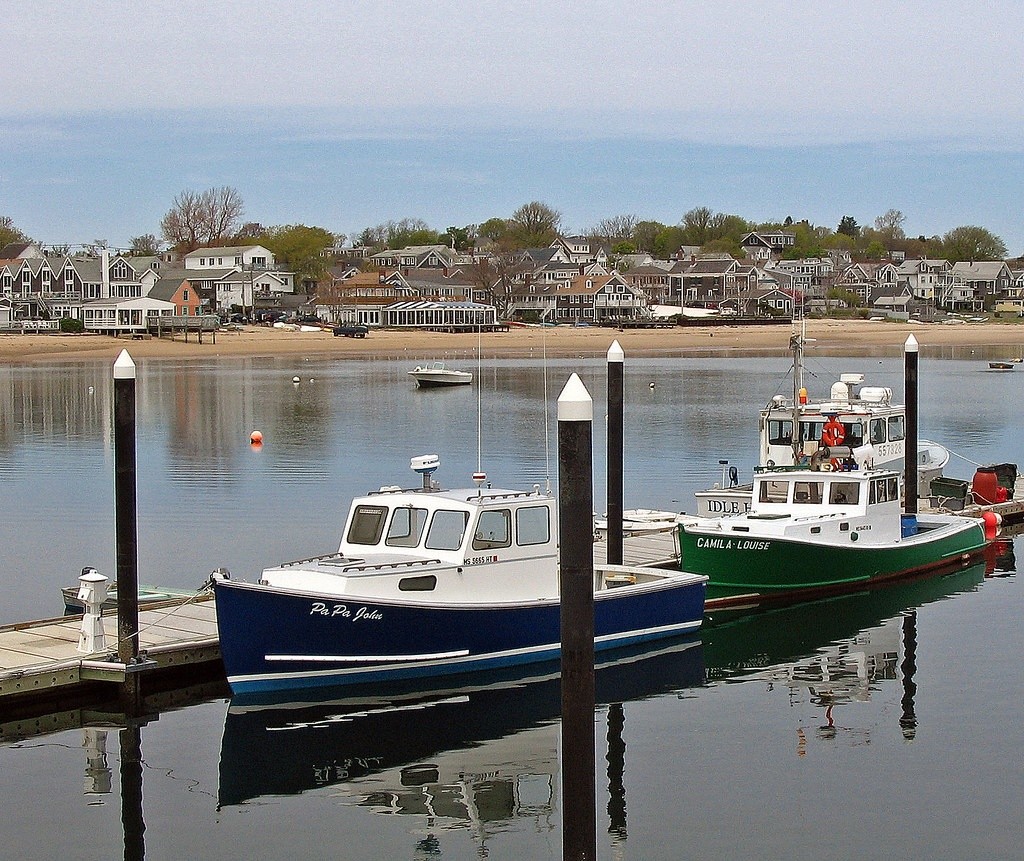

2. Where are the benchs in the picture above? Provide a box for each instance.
[139,593,169,600]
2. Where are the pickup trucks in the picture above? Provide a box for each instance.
[333,321,369,339]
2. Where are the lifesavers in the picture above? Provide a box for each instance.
[821,422,845,446]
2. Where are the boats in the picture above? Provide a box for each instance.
[407,361,474,387]
[209,306,710,699]
[671,332,1002,617]
[692,275,950,523]
[989,362,1014,369]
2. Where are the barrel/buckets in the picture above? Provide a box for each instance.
[901,514,918,539]
[973,466,998,505]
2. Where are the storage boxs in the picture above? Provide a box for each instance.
[929,476,969,498]
[929,494,966,511]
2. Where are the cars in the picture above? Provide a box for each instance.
[220,309,323,328]
